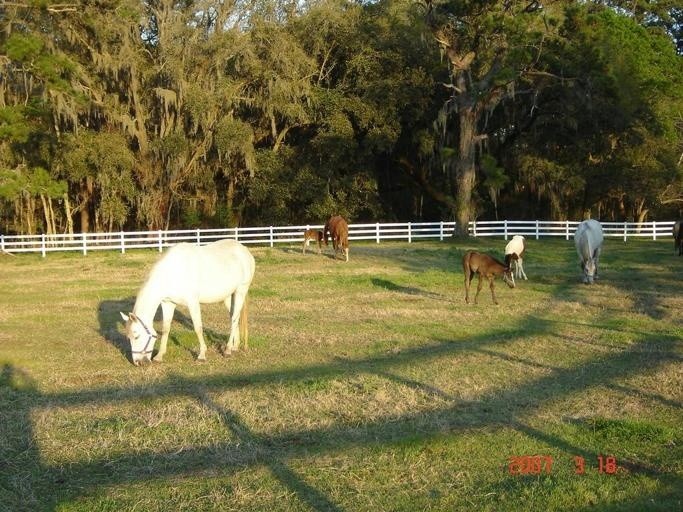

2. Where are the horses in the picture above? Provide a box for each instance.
[573,218,605,285]
[462,251,515,308]
[672,219,683,258]
[302,229,328,255]
[118,238,256,367]
[504,234,529,284]
[324,215,350,263]
